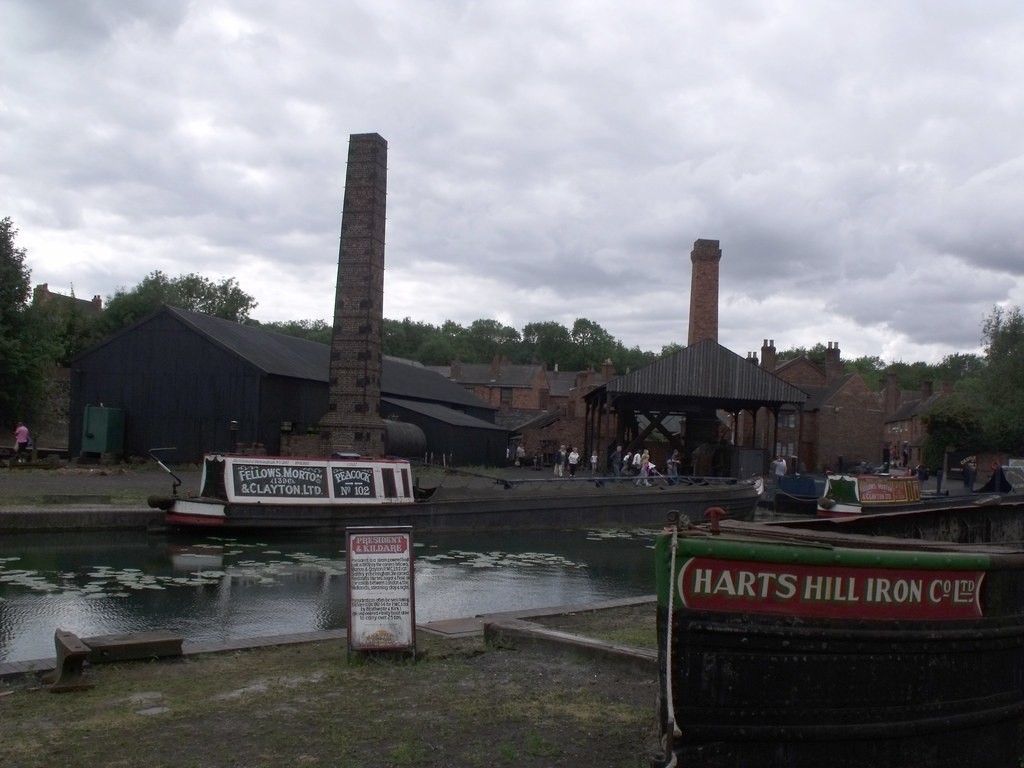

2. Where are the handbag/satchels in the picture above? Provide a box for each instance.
[553,463,558,475]
[514,457,520,466]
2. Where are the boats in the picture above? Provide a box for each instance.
[143,447,764,536]
[845,496,847,499]
[776,474,1024,516]
[647,500,1024,747]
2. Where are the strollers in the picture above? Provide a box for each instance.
[649,466,668,489]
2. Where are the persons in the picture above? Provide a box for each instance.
[890,444,944,494]
[610,445,680,487]
[772,454,787,488]
[590,450,598,475]
[516,442,525,469]
[13,423,29,463]
[974,462,1012,494]
[554,445,579,478]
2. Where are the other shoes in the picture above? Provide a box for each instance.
[573,474,576,478]
[645,483,652,487]
[570,473,572,476]
